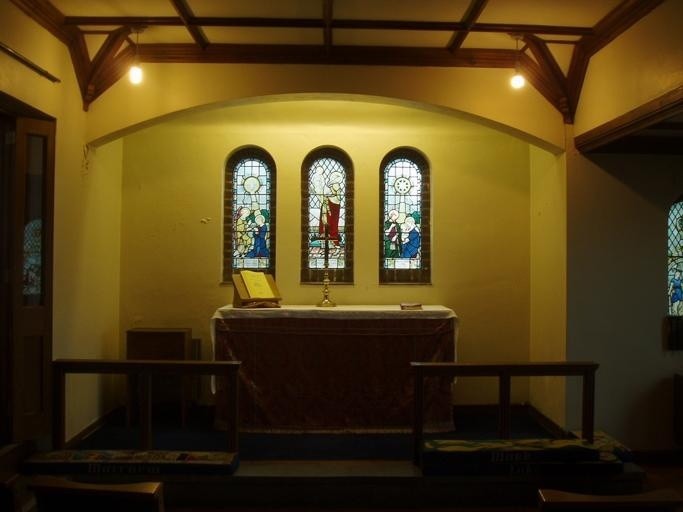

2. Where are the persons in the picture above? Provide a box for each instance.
[667,271,683,316]
[400,216,420,258]
[318,171,344,248]
[232,206,270,258]
[384,210,402,258]
[400,223,410,244]
[311,167,326,194]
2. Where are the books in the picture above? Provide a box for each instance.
[239,270,275,299]
[399,303,423,310]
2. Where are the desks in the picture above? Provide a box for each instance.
[211,303,460,440]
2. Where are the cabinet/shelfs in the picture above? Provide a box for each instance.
[122,328,194,429]
